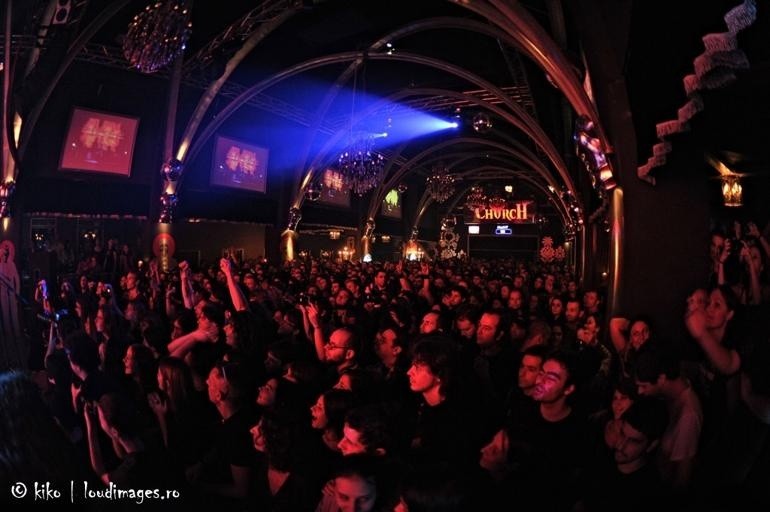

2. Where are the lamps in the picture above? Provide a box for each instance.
[446,107,463,132]
[336,58,385,200]
[425,152,454,207]
[465,184,488,212]
[489,188,507,212]
[287,208,304,233]
[720,169,749,210]
[360,218,377,242]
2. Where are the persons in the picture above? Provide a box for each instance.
[1,217,770,511]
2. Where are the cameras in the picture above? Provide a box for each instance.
[101,288,112,298]
[55,308,74,321]
[298,294,311,305]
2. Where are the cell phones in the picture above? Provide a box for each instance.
[89,407,94,416]
